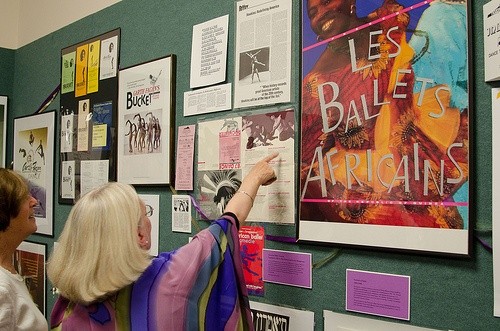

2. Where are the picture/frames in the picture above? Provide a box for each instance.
[12,109,57,238]
[0,94,9,168]
[12,240,48,321]
[114,53,177,189]
[297,0,476,262]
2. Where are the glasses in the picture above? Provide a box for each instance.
[144,204,154,218]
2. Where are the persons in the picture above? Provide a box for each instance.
[125,112,162,153]
[303,0,468,230]
[245,51,265,82]
[46,151,280,331]
[0,168,48,331]
[241,112,295,147]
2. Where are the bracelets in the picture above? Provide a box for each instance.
[238,190,254,207]
